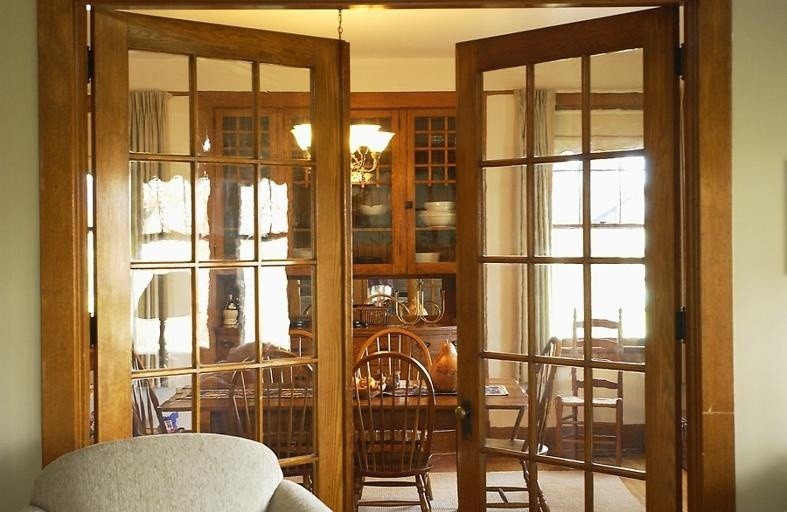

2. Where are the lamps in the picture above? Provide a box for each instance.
[290,123,395,189]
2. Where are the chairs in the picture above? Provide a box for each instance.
[353,351,434,512]
[262,328,316,354]
[353,328,433,367]
[228,352,315,487]
[554,306,624,467]
[131,349,191,434]
[482,335,563,512]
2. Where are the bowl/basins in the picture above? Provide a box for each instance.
[293,248,312,258]
[359,204,390,216]
[418,211,458,228]
[352,389,386,400]
[422,201,457,212]
[290,320,308,328]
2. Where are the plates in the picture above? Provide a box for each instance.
[353,257,383,264]
[355,215,391,228]
[415,252,441,263]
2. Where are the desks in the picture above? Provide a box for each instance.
[155,375,530,467]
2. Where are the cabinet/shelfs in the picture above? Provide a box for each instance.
[197,92,486,371]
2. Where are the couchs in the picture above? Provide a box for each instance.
[16,432,336,512]
